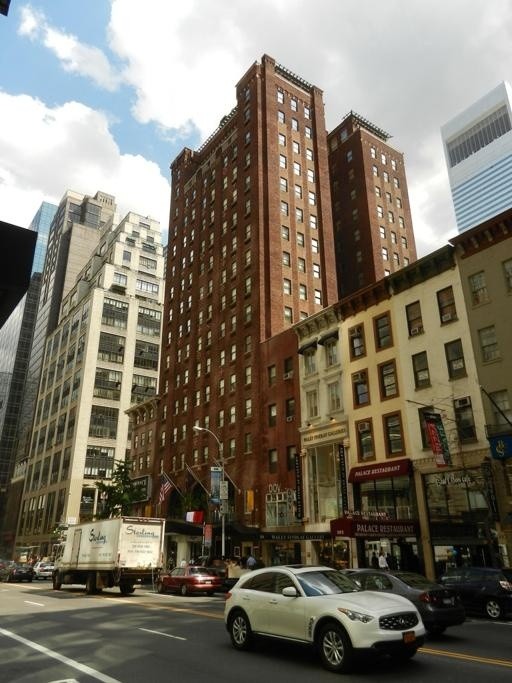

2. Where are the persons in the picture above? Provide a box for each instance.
[372,551,378,568]
[257,555,265,568]
[189,557,196,564]
[246,554,256,569]
[180,558,187,566]
[377,552,388,570]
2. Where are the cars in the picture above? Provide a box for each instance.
[0,560,54,582]
[223,564,426,674]
[157,566,222,597]
[336,568,512,633]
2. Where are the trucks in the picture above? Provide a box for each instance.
[52,515,166,595]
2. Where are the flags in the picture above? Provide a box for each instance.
[183,467,208,525]
[158,469,173,505]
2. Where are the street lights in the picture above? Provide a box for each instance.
[193,426,225,563]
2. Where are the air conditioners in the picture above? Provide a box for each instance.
[349,326,361,337]
[410,326,419,335]
[282,372,293,380]
[285,414,293,422]
[356,421,369,432]
[351,372,366,383]
[456,396,470,408]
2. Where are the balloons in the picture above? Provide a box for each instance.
[451,549,457,556]
[448,551,452,556]
